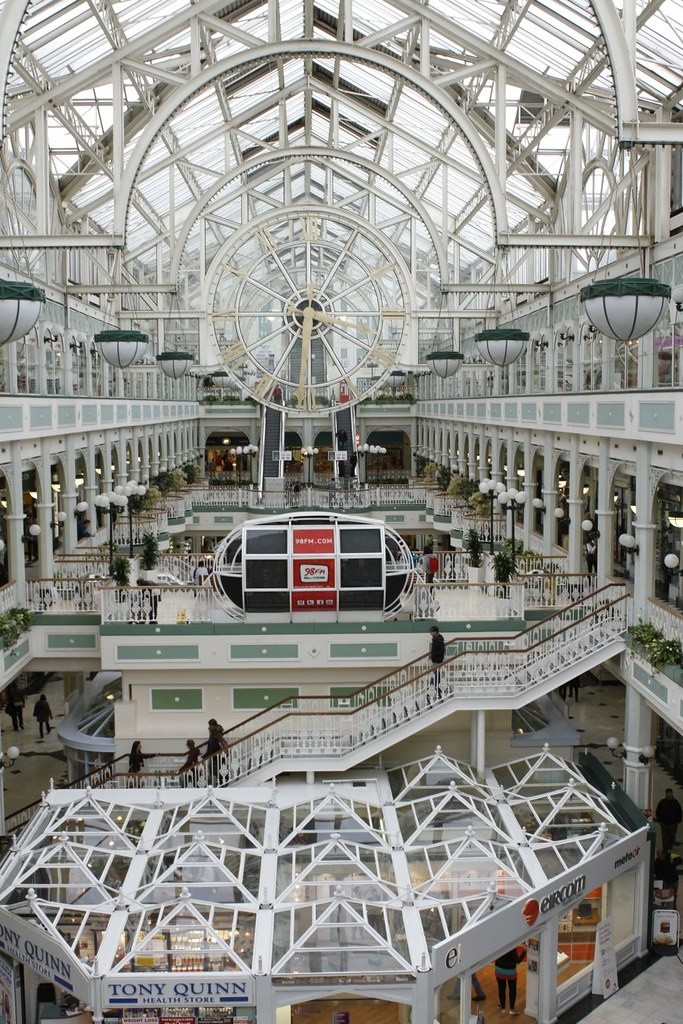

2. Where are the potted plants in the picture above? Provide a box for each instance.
[109,556,131,603]
[204,395,259,405]
[465,529,484,590]
[488,549,519,621]
[360,394,417,404]
[139,532,161,570]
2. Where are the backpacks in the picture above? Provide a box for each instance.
[210,733,231,759]
[426,555,438,573]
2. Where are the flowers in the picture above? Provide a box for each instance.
[628,615,683,684]
[0,607,37,657]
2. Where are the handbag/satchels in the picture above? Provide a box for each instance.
[5,706,10,714]
[33,702,39,716]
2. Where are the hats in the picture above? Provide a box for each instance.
[430,626,439,631]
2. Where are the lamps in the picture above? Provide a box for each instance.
[0,141,671,391]
[0,448,683,575]
[639,746,655,765]
[606,736,627,759]
[0,746,20,770]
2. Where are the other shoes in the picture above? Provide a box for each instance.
[497,1005,505,1014]
[20,725,24,729]
[14,729,18,731]
[473,995,486,1001]
[448,996,459,999]
[508,1010,520,1014]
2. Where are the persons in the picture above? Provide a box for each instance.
[338,452,357,477]
[558,486,569,516]
[2,362,32,390]
[494,946,527,1015]
[585,539,597,577]
[176,740,200,788]
[130,577,161,623]
[399,541,439,601]
[336,427,348,451]
[33,694,53,738]
[274,385,282,403]
[207,560,221,574]
[655,788,683,850]
[654,848,679,911]
[200,719,229,788]
[193,559,208,596]
[0,680,25,733]
[211,450,235,472]
[559,677,578,702]
[447,973,487,1001]
[291,474,301,503]
[584,492,590,512]
[128,741,145,789]
[427,626,446,701]
[487,371,494,387]
